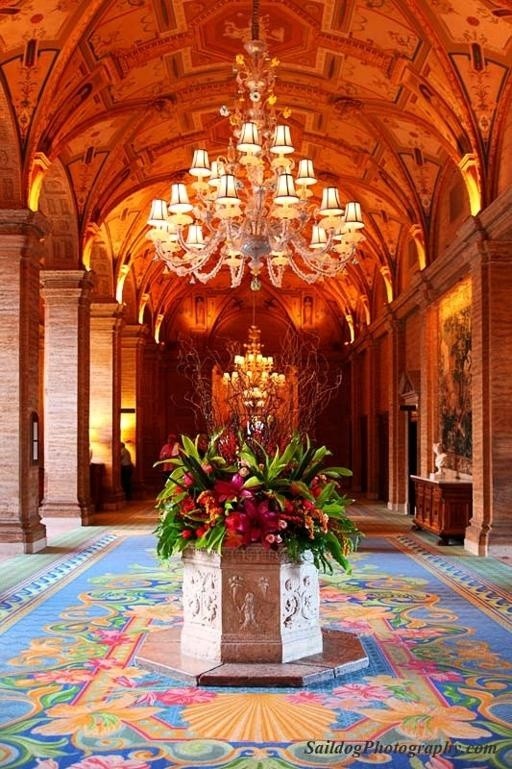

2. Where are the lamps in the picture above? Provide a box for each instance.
[219,284,287,415]
[141,1,375,295]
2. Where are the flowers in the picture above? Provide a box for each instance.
[141,424,367,579]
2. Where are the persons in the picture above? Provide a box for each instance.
[120,442,132,501]
[432,443,448,468]
[159,432,179,483]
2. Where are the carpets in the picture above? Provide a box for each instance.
[0,516,512,768]
[127,495,386,522]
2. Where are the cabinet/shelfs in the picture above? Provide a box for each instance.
[406,469,473,548]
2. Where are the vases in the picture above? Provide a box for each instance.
[172,537,328,666]
